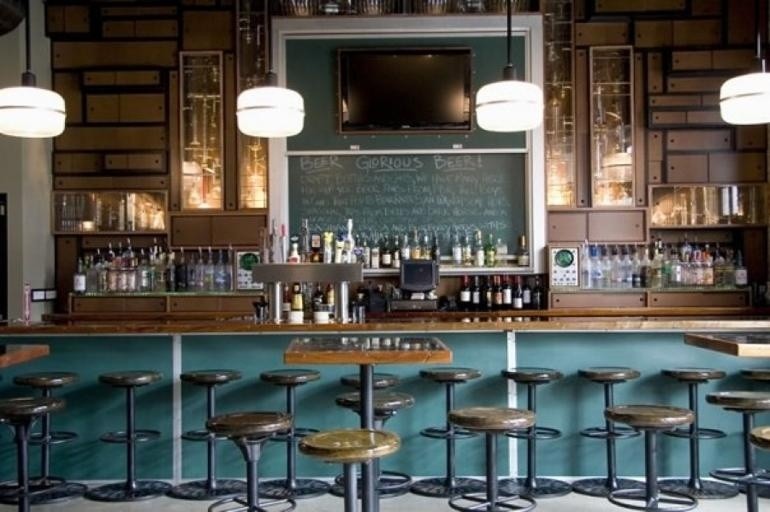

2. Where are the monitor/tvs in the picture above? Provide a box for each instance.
[400,259,437,290]
[331,45,473,136]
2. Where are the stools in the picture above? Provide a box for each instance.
[9,372,85,502]
[497,366,572,501]
[608,406,697,512]
[329,392,415,501]
[658,368,738,503]
[735,368,770,386]
[165,371,249,499]
[338,372,398,391]
[85,372,173,504]
[449,408,535,512]
[2,396,68,511]
[572,368,648,499]
[203,411,290,511]
[751,425,770,444]
[409,368,489,498]
[259,372,331,501]
[706,391,770,512]
[298,430,400,511]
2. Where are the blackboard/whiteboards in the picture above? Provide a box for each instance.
[287,153,528,257]
[284,35,527,152]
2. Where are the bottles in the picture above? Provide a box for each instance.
[703,250,713,287]
[283,286,291,321]
[300,219,310,251]
[601,248,611,287]
[421,233,432,260]
[631,248,639,288]
[713,243,735,288]
[187,255,195,290]
[392,235,401,269]
[621,248,631,287]
[453,236,462,266]
[459,275,471,312]
[663,237,703,287]
[652,248,663,289]
[411,230,421,260]
[432,232,440,265]
[611,248,621,287]
[353,293,365,325]
[486,234,495,267]
[154,254,166,291]
[311,230,321,262]
[361,238,370,268]
[735,250,748,288]
[512,275,522,311]
[215,252,226,289]
[381,236,391,267]
[591,248,601,288]
[204,253,215,289]
[291,284,303,311]
[279,224,289,263]
[493,276,503,312]
[86,255,97,292]
[463,237,472,266]
[471,276,482,312]
[503,276,512,312]
[518,235,529,267]
[580,245,591,289]
[474,231,485,267]
[522,275,532,311]
[269,220,279,262]
[97,243,160,292]
[532,276,543,311]
[482,275,493,312]
[353,235,361,263]
[177,253,187,291]
[313,282,323,305]
[400,234,410,259]
[74,257,85,294]
[195,252,204,290]
[326,284,334,304]
[371,245,381,268]
[166,253,177,291]
[639,248,652,288]
[323,226,334,263]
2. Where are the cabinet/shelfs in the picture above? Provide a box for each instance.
[40,0,275,326]
[545,0,769,323]
[275,0,541,19]
[275,269,543,325]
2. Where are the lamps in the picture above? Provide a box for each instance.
[234,0,305,141]
[0,0,67,139]
[475,0,549,134]
[717,58,769,127]
